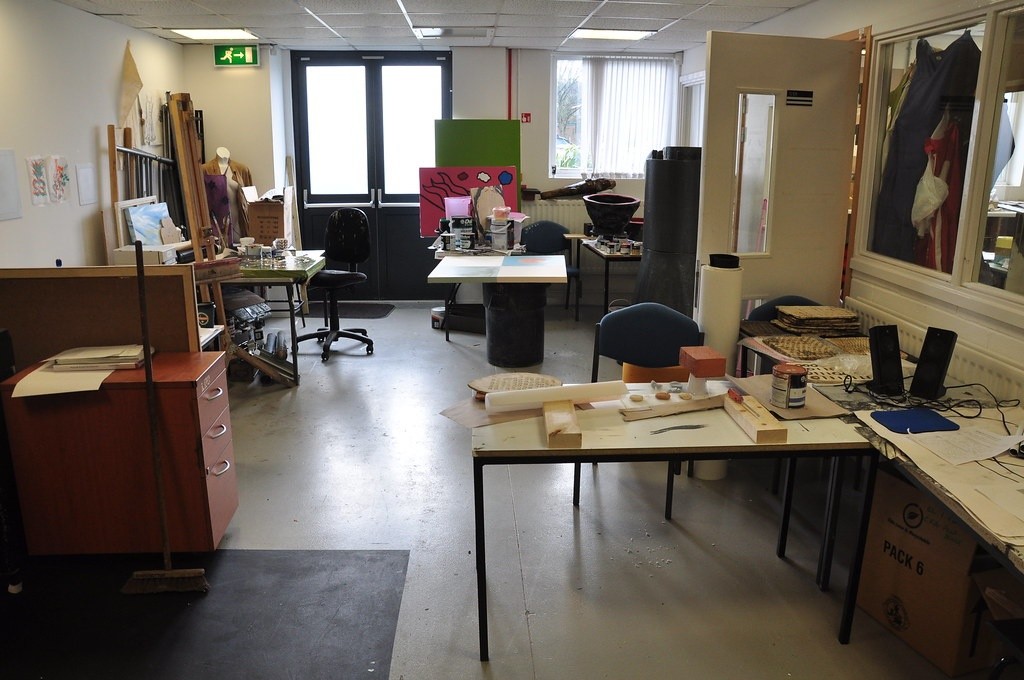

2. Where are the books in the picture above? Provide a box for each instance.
[54,344,155,370]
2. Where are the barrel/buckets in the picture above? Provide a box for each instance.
[490,219,514,250]
[440,234,455,251]
[460,233,475,249]
[431,307,446,329]
[450,216,473,250]
[443,195,473,220]
[488,210,531,243]
[770,364,808,410]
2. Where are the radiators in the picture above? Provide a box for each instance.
[522,199,641,277]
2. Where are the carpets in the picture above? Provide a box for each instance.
[293,301,395,319]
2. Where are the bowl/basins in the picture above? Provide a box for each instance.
[492,206,511,221]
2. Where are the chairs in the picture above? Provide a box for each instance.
[567,302,700,518]
[288,208,373,360]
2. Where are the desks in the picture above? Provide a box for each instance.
[742,324,1023,676]
[471,377,882,662]
[220,253,325,385]
[427,252,567,366]
[574,237,640,317]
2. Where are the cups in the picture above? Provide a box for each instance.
[242,255,286,268]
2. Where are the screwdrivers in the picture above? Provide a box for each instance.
[728,388,760,417]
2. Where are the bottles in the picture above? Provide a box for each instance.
[621,245,630,254]
[632,244,640,255]
[597,240,614,254]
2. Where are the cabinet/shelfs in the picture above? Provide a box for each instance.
[2,348,238,556]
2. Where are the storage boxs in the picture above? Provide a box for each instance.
[247,198,285,244]
[853,465,1020,678]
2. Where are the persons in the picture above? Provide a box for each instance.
[200,146,255,244]
